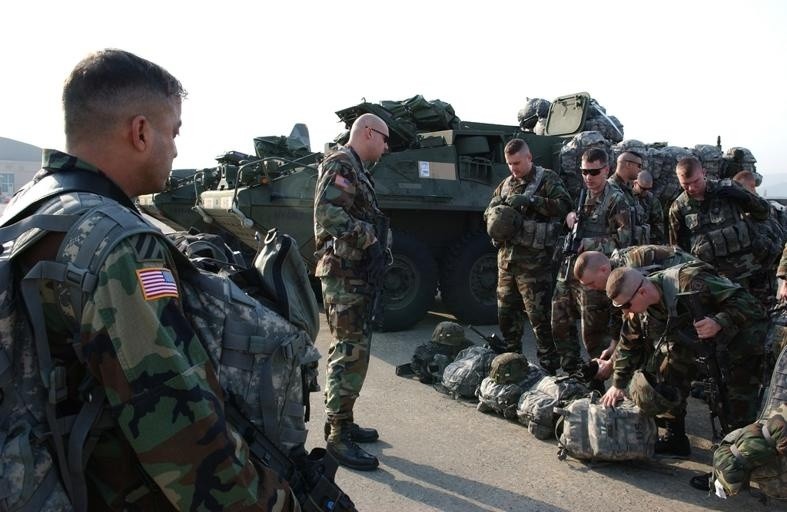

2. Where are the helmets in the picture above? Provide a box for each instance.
[486,205,523,241]
[626,369,682,416]
[431,320,465,347]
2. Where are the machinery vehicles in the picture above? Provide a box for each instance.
[134,151,257,261]
[190,92,762,332]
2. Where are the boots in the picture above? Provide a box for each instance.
[325,411,379,471]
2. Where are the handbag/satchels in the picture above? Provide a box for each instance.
[742,213,785,267]
[609,140,762,201]
[518,97,549,128]
[559,131,614,198]
[585,97,623,142]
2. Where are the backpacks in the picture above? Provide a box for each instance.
[396,336,657,466]
[760,297,787,386]
[0,193,133,512]
[50,201,322,512]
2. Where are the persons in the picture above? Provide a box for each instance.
[314,114,392,470]
[0,49,301,512]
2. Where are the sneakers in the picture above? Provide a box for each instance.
[654,433,691,459]
[690,473,717,492]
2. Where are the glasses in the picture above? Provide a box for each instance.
[580,165,607,176]
[365,126,389,143]
[608,279,643,312]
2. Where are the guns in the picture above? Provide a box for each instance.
[351,218,390,335]
[563,181,586,258]
[675,291,730,439]
[224,403,357,512]
[467,325,511,353]
[556,362,599,384]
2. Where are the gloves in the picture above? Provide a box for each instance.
[488,195,507,208]
[365,242,385,279]
[508,194,528,208]
